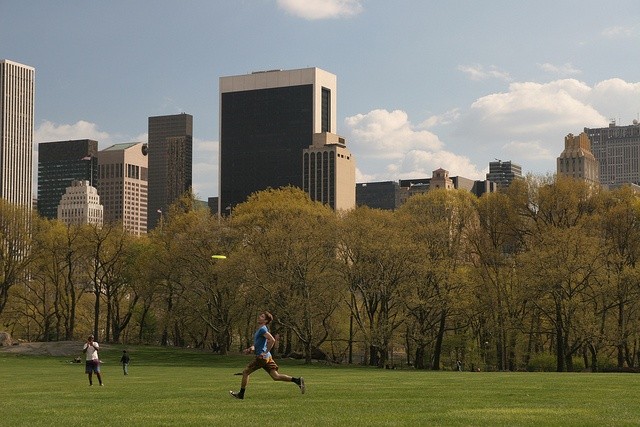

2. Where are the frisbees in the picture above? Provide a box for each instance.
[211,254,226,260]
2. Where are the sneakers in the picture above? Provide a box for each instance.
[297,376,305,394]
[229,390,244,401]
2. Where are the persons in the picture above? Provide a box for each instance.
[83,334,104,387]
[120,349,130,375]
[229,312,305,399]
